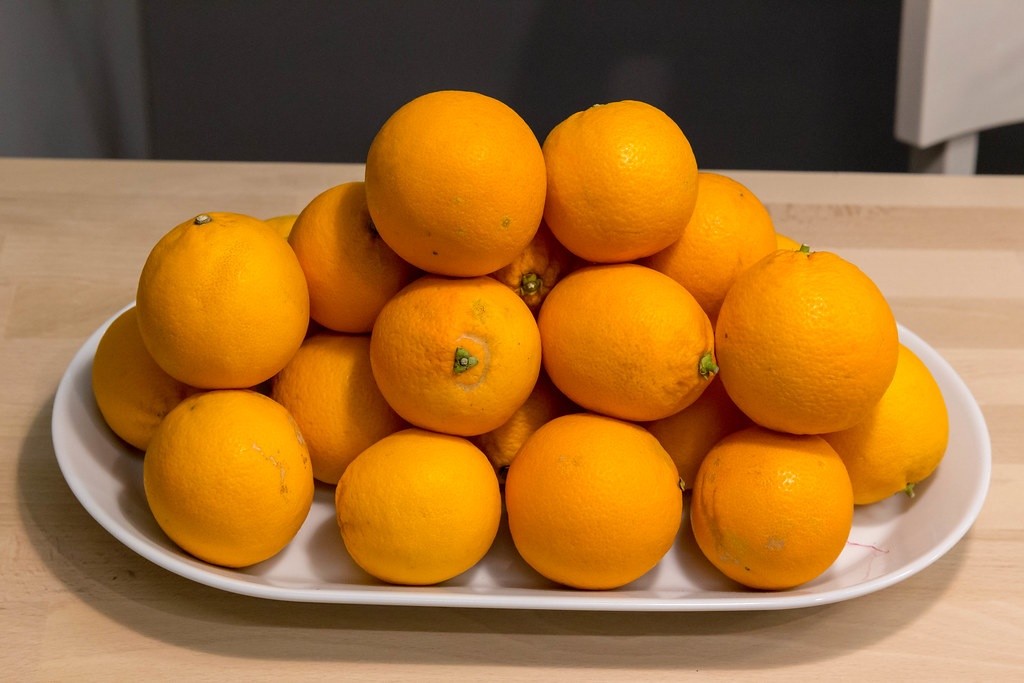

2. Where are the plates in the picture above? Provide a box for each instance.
[51,280,991,612]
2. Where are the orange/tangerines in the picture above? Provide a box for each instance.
[89,91,948,590]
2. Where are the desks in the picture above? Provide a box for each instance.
[0,160,1024,683]
